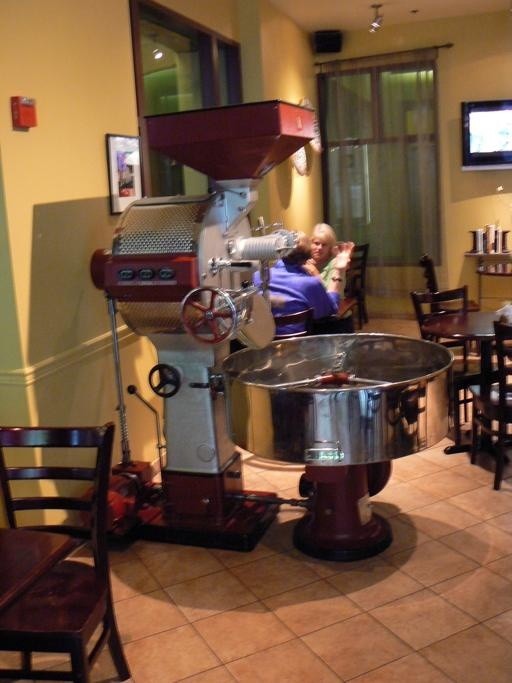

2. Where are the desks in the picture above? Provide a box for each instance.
[0,527,75,607]
[463,249,512,310]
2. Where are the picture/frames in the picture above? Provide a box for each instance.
[103,131,148,217]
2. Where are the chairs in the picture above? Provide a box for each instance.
[0,420,133,682]
[269,242,370,341]
[409,283,512,492]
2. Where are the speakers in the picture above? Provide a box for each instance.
[315,31,342,53]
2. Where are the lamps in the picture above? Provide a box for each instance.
[366,3,386,34]
[147,30,165,62]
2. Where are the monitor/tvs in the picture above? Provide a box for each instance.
[461,99,512,172]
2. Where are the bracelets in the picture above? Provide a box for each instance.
[330,276,346,281]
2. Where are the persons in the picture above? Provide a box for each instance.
[252,228,352,342]
[298,220,336,292]
